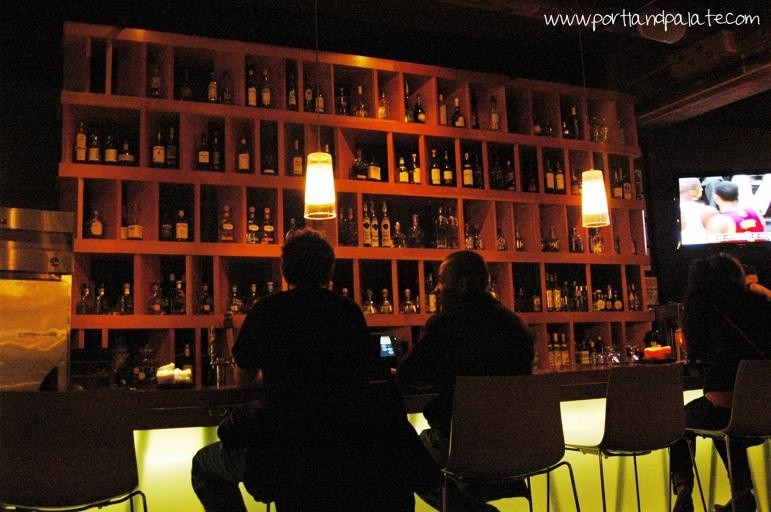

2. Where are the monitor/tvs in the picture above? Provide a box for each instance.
[672,170,771,252]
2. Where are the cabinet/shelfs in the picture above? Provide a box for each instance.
[58,18,660,393]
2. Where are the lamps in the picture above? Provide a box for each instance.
[581,22,612,229]
[302,3,341,224]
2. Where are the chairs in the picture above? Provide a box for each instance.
[1,390,150,512]
[563,358,713,512]
[417,370,585,512]
[680,359,771,511]
[269,376,417,510]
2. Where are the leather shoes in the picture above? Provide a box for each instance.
[673,488,694,512]
[713,493,756,512]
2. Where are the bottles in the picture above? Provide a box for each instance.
[391,222,406,246]
[147,282,166,314]
[630,283,638,308]
[369,202,380,247]
[246,206,258,245]
[261,68,272,105]
[363,290,375,313]
[487,277,501,297]
[287,73,298,108]
[166,127,176,166]
[404,80,413,123]
[352,85,369,116]
[96,284,111,314]
[601,118,610,143]
[411,153,421,184]
[436,207,448,247]
[148,61,162,97]
[128,204,145,240]
[597,336,605,364]
[606,285,614,309]
[246,283,258,308]
[346,208,357,244]
[594,290,603,310]
[473,92,480,128]
[546,120,556,135]
[612,171,621,199]
[379,90,391,118]
[505,161,517,189]
[171,281,184,312]
[474,155,484,187]
[569,225,580,252]
[415,93,427,123]
[249,68,257,106]
[535,116,543,135]
[555,271,561,311]
[575,285,582,309]
[532,293,541,310]
[408,214,423,247]
[555,159,564,193]
[120,202,129,240]
[105,137,117,162]
[175,210,188,241]
[179,66,195,99]
[303,69,314,111]
[430,149,442,186]
[118,141,134,163]
[219,204,236,242]
[489,95,501,130]
[562,331,570,367]
[570,104,581,137]
[614,289,621,309]
[315,80,326,111]
[120,283,134,313]
[89,132,101,163]
[168,274,175,295]
[543,157,555,193]
[472,223,481,247]
[87,211,104,239]
[579,339,589,364]
[591,114,599,139]
[446,209,461,248]
[237,125,252,172]
[261,136,280,175]
[395,155,408,183]
[338,207,348,242]
[426,274,438,314]
[75,121,87,162]
[212,133,222,169]
[516,287,530,309]
[443,151,453,184]
[439,94,448,125]
[368,154,382,181]
[198,282,211,313]
[151,132,164,164]
[571,162,579,194]
[206,71,218,102]
[546,273,554,311]
[339,86,348,114]
[181,344,195,370]
[351,149,367,178]
[401,289,418,313]
[562,120,570,139]
[465,224,475,247]
[452,97,465,127]
[555,333,562,367]
[340,288,349,297]
[584,286,588,308]
[515,225,524,249]
[230,286,243,312]
[492,157,504,187]
[462,151,473,187]
[363,201,372,245]
[196,133,210,166]
[546,333,554,367]
[379,288,392,313]
[325,143,331,153]
[290,134,304,173]
[617,122,626,142]
[80,283,96,314]
[264,281,275,294]
[261,206,275,243]
[222,70,234,103]
[563,280,571,309]
[159,212,173,238]
[589,341,596,364]
[380,200,394,247]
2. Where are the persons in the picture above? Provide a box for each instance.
[394,250,536,512]
[679,177,720,246]
[669,254,771,512]
[705,179,765,243]
[192,233,380,512]
